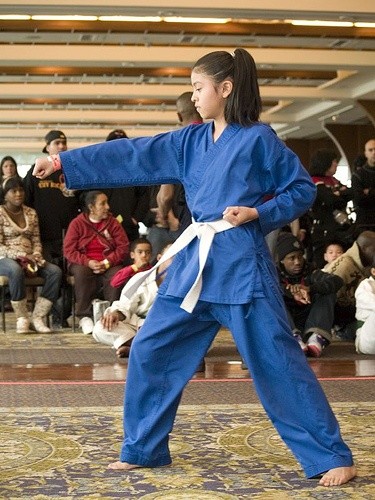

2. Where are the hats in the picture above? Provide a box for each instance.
[42,130,66,153]
[273,237,304,263]
[2,177,24,196]
[106,130,128,142]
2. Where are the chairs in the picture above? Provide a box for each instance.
[0,228,76,333]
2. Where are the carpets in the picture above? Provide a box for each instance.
[0,375,375,500]
[0,311,375,362]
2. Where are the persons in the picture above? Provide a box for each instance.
[263,134,375,358]
[0,92,249,379]
[31,47,358,487]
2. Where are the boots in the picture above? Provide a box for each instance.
[10,297,53,334]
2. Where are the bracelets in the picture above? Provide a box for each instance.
[48,154,61,172]
[103,259,110,270]
[130,264,138,272]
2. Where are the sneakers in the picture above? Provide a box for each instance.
[292,332,326,357]
[79,316,94,335]
